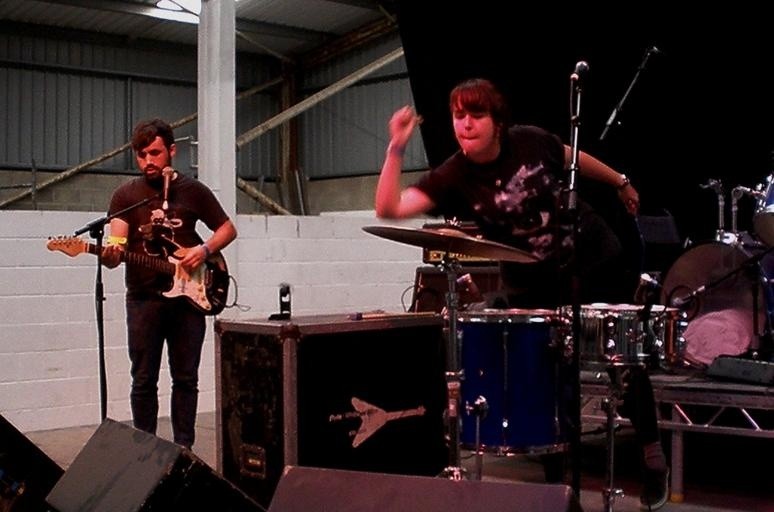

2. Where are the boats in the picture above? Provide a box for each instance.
[45,417,264,512]
[0,412,65,512]
[264,463,577,511]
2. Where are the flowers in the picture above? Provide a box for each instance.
[162,167,175,200]
[575,61,591,89]
[646,38,659,56]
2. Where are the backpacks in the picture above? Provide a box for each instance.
[755,203,774,247]
[567,300,647,373]
[647,304,688,375]
[409,265,507,375]
[651,233,770,370]
[454,306,578,460]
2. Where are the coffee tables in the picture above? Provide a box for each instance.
[361,225,542,262]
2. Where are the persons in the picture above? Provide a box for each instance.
[96,116,240,453]
[370,75,676,512]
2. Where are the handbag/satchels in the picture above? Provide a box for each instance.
[200,244,210,258]
[618,173,631,191]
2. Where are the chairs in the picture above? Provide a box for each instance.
[638,464,670,511]
[544,455,569,484]
